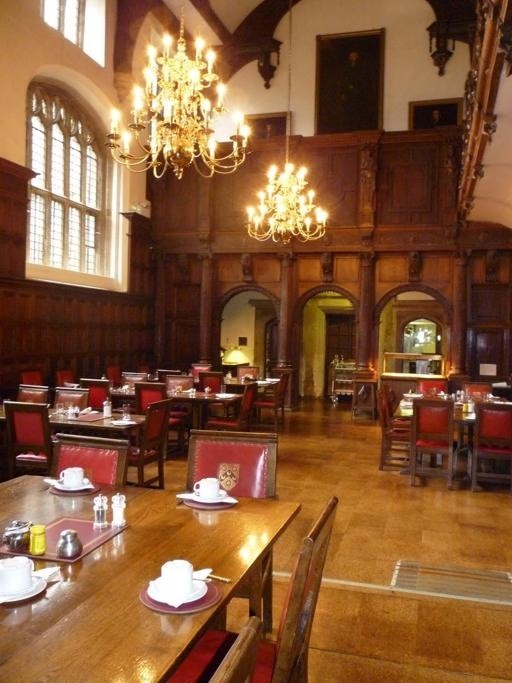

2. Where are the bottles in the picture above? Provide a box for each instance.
[442,389,475,415]
[4,521,32,553]
[103,398,112,417]
[28,525,46,555]
[56,529,82,559]
[93,493,108,529]
[111,492,127,526]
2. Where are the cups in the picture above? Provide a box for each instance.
[194,478,220,496]
[123,403,130,420]
[170,385,212,398]
[160,560,194,585]
[52,401,80,420]
[60,467,84,486]
[482,392,489,401]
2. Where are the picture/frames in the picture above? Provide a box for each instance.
[244,111,293,139]
[314,29,384,136]
[408,97,463,130]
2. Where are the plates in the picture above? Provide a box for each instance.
[400,402,414,408]
[55,480,87,492]
[265,378,280,382]
[255,381,271,384]
[147,578,207,606]
[192,490,227,503]
[493,401,512,405]
[403,393,422,397]
[90,411,100,414]
[217,394,235,399]
[2,578,48,603]
[113,421,133,426]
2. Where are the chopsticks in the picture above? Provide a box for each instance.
[208,574,230,582]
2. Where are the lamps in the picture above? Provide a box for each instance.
[246,0,328,245]
[254,36,283,89]
[425,19,458,76]
[105,0,254,180]
